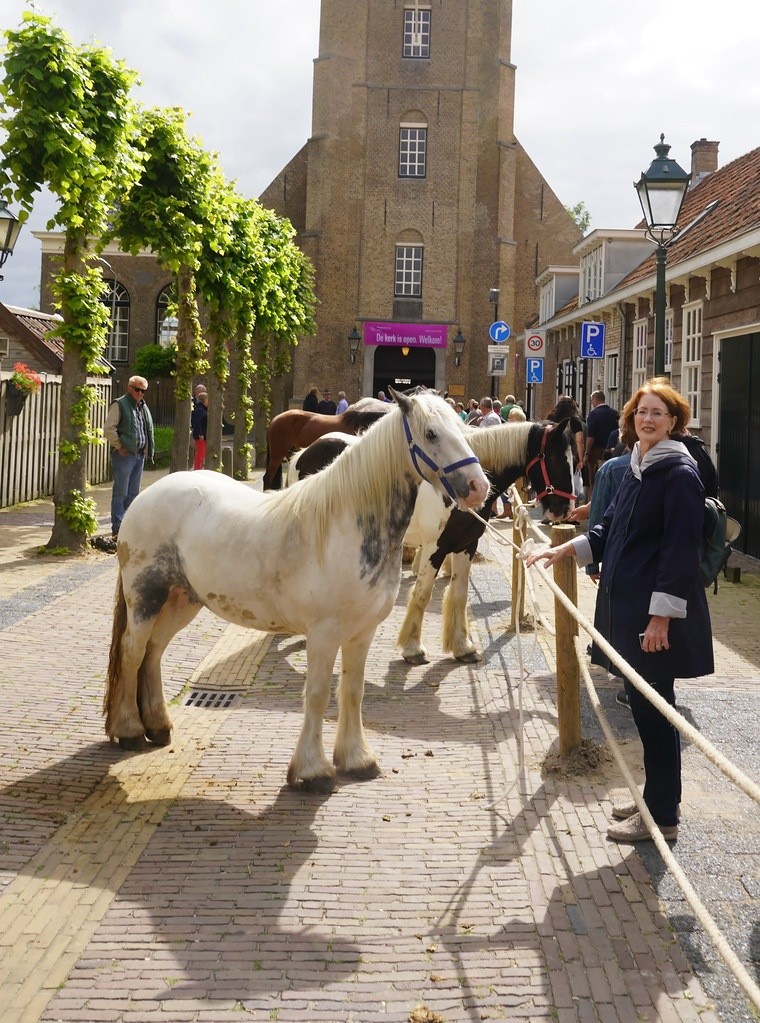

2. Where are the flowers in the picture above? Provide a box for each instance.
[10,362,42,397]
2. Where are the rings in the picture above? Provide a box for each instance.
[656,645,660,648]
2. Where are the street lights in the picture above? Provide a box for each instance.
[633,133,694,378]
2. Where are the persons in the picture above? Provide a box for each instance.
[447,393,534,519]
[319,389,336,415]
[191,385,207,469]
[585,454,632,709]
[541,389,620,526]
[105,376,155,539]
[679,428,717,498]
[378,391,391,402]
[303,387,318,413]
[335,391,348,414]
[522,383,714,841]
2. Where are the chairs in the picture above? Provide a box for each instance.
[712,516,743,595]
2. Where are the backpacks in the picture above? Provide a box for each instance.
[664,463,742,594]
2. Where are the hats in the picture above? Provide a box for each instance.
[321,389,332,394]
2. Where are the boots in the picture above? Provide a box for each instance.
[497,502,514,518]
[490,501,499,517]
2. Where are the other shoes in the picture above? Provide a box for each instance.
[616,690,631,709]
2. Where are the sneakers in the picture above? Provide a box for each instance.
[612,800,681,819]
[607,811,678,840]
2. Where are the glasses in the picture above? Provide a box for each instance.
[632,406,670,419]
[129,385,146,394]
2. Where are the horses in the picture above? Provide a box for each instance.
[100,385,576,792]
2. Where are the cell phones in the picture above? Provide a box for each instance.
[639,633,645,649]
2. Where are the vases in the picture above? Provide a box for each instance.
[5,381,28,416]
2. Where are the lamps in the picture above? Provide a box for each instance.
[0,200,27,282]
[348,326,362,365]
[452,328,466,367]
[401,346,410,357]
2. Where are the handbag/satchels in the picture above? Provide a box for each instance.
[574,468,585,500]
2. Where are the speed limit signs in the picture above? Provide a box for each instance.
[524,328,548,358]
